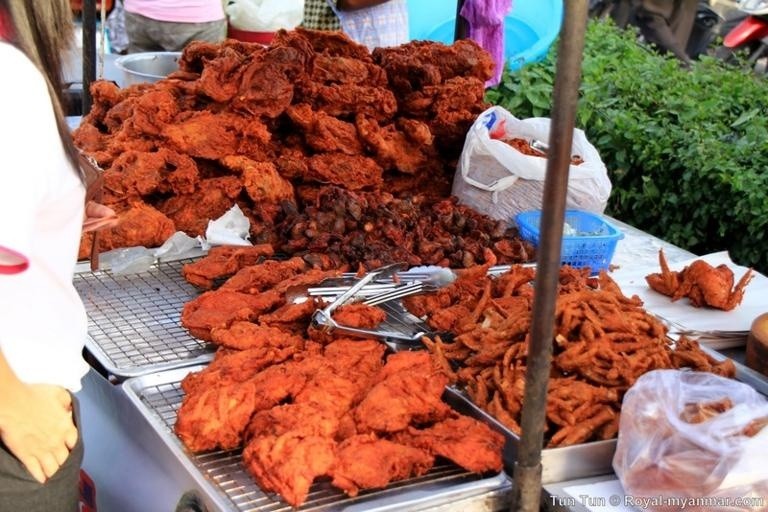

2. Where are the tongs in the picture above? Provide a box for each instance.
[309,261,455,348]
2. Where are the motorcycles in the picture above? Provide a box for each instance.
[709,0,768,65]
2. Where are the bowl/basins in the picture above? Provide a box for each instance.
[400,0,564,70]
[113,52,182,88]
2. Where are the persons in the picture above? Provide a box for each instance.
[0,0,94,512]
[105,0,511,86]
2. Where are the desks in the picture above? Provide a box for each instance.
[67,112,768,512]
